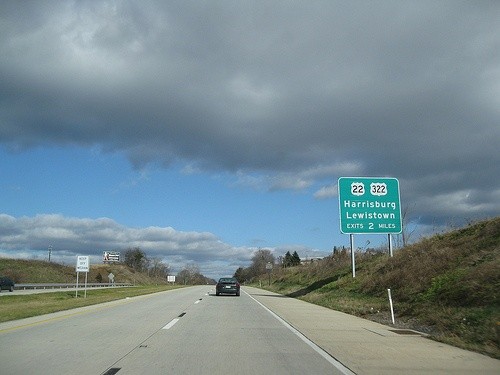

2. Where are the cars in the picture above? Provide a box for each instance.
[215,278,241,296]
